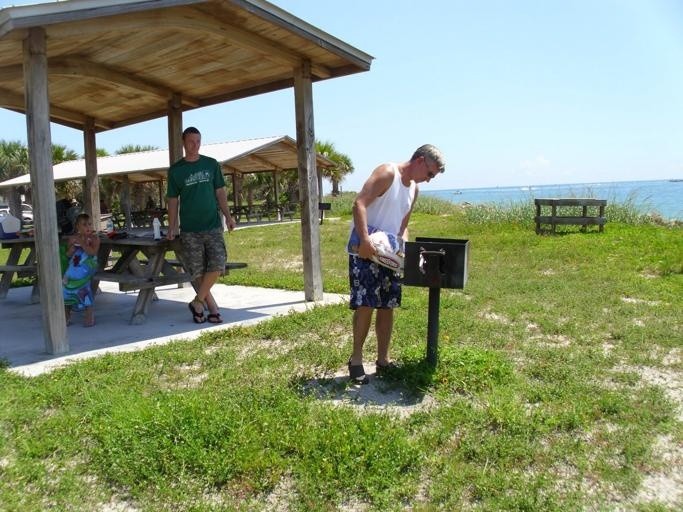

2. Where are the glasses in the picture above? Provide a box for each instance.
[424,160,435,178]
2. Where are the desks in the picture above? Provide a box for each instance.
[60,235,180,326]
[106,205,168,229]
[1,233,42,302]
[535,197,607,234]
[231,203,300,224]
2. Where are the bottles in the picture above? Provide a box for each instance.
[152,218,160,240]
[106,218,112,234]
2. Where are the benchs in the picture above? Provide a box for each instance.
[1,265,40,303]
[88,256,248,325]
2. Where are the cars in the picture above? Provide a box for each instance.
[0,203,33,225]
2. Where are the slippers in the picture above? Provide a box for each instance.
[208,314,223,322]
[189,303,206,323]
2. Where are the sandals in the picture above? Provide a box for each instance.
[376,360,397,368]
[349,356,368,384]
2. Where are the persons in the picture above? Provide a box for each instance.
[165,126,235,323]
[62,213,100,326]
[145,196,155,209]
[343,142,446,385]
[53,190,109,242]
